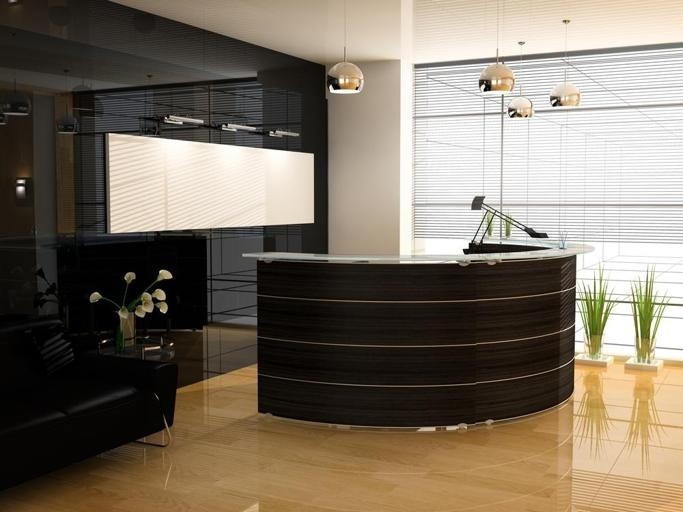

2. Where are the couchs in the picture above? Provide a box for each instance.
[0,319,176,490]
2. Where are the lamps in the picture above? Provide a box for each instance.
[326,1,363,94]
[0,67,163,138]
[476,0,581,120]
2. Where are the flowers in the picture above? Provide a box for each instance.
[31,265,60,309]
[88,268,175,320]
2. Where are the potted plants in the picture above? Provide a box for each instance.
[572,259,673,375]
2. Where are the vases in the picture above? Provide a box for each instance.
[118,313,135,347]
